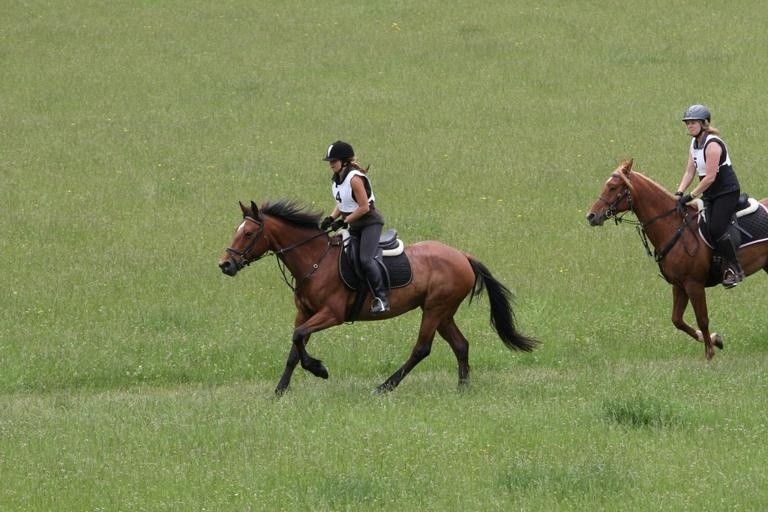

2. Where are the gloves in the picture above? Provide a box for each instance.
[321,216,346,232]
[674,191,694,215]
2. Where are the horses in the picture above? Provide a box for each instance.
[585,157,768,365]
[218,194,544,401]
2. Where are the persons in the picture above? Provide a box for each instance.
[321,140,391,317]
[673,104,744,287]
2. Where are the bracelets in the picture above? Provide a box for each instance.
[675,192,683,196]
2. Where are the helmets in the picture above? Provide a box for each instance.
[682,104,711,121]
[322,140,354,161]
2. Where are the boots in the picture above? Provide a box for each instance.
[361,257,388,314]
[716,231,742,289]
